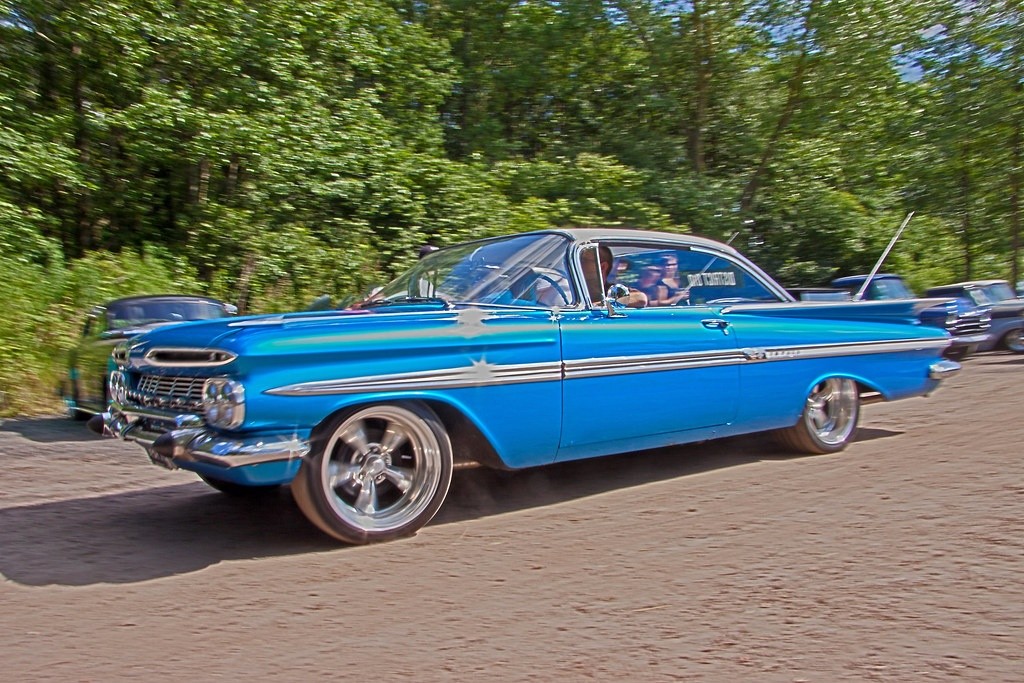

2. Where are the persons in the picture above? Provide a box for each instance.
[531,245,649,311]
[635,256,692,307]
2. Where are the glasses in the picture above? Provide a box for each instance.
[580,256,597,266]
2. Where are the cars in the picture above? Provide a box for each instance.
[86,229,964,545]
[831,273,1024,359]
[67,293,238,424]
